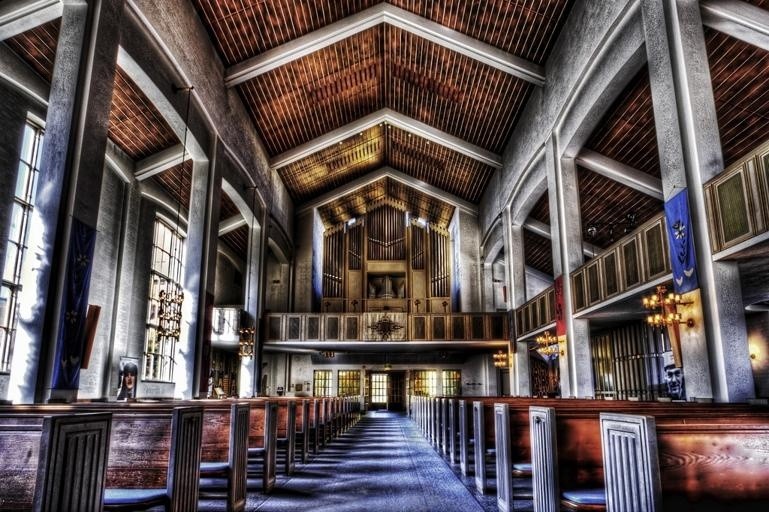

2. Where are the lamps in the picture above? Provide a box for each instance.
[157,83,195,343]
[642,286,696,328]
[237,186,258,360]
[493,350,512,369]
[536,331,565,356]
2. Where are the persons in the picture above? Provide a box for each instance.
[664,364,683,400]
[118,362,138,397]
